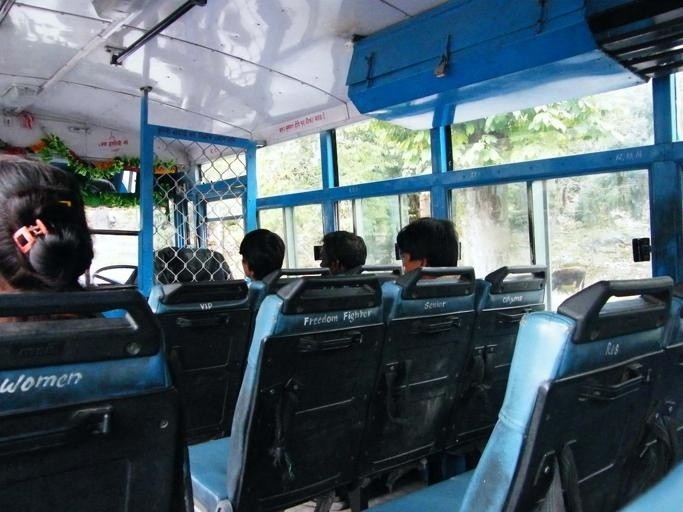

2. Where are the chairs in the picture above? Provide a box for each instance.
[1,285,197,512]
[152,265,549,512]
[363,275,682,511]
[131,246,233,282]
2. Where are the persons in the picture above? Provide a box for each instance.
[303,218,460,511]
[319,229,369,279]
[2,154,97,323]
[240,230,286,291]
